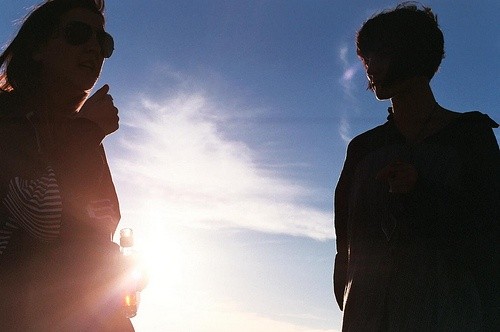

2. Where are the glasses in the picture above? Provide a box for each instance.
[58,22,114,58]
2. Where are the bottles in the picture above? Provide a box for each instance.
[119,228,139,319]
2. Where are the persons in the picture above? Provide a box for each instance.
[0,0,136,331]
[332,1,500,332]
